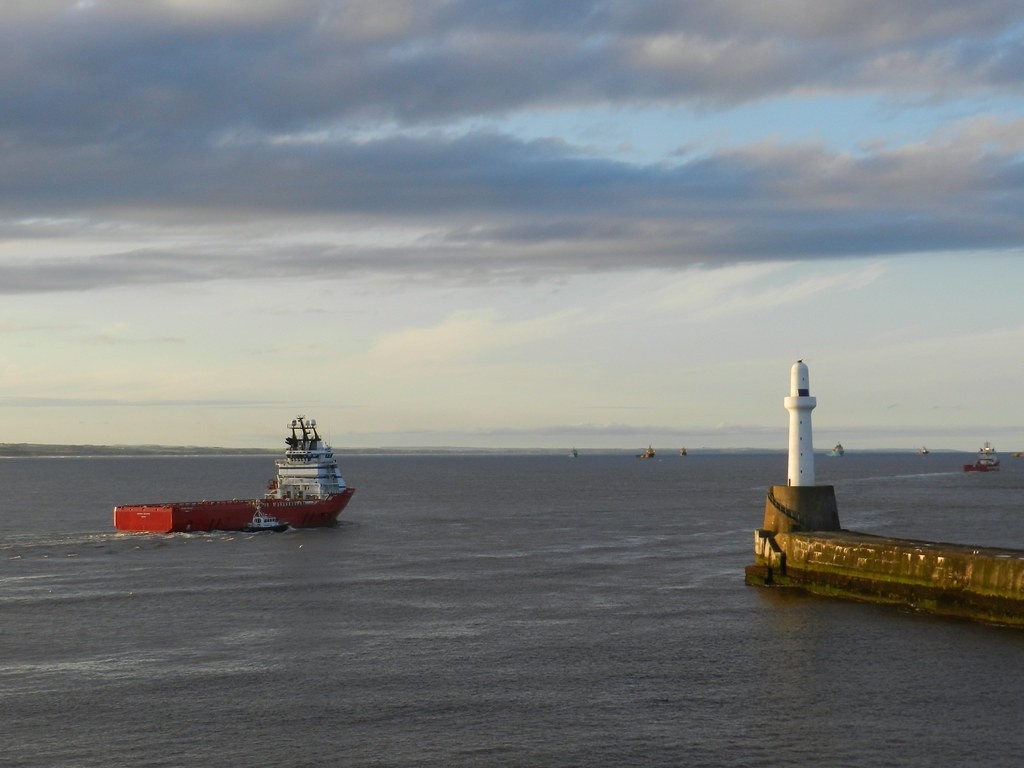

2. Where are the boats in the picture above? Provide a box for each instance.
[960,443,1001,472]
[113,414,356,532]
